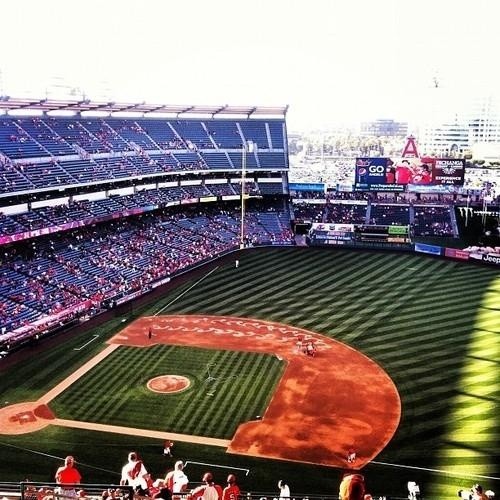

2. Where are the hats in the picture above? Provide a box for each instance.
[202,473,212,482]
[227,475,236,483]
[128,453,136,460]
[65,455,74,466]
[174,461,183,470]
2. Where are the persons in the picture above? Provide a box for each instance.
[471,483,489,500]
